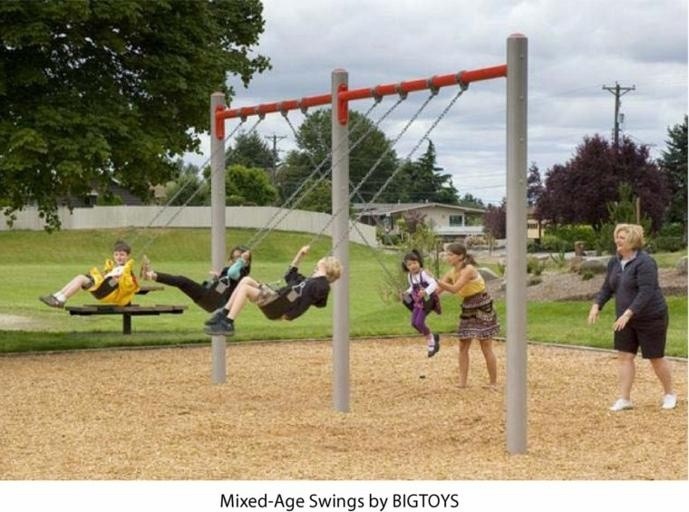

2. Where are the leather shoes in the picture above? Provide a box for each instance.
[427,333,440,356]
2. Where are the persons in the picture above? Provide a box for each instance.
[400,243,503,389]
[587,222,677,412]
[141,243,344,336]
[39,239,141,308]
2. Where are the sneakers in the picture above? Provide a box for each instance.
[139,255,150,281]
[202,308,235,336]
[610,398,634,411]
[38,294,65,309]
[662,391,678,410]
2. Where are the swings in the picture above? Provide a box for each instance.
[84,105,265,300]
[258,73,468,322]
[195,80,409,313]
[280,93,438,314]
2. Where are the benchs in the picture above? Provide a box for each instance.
[65,304,188,336]
